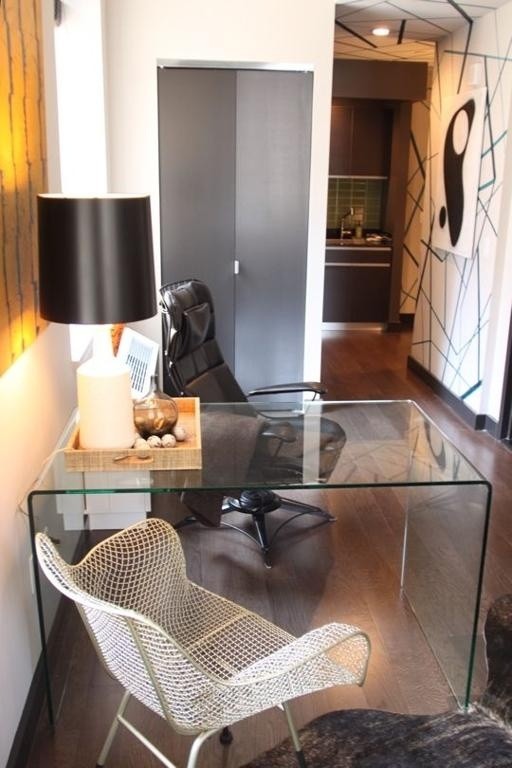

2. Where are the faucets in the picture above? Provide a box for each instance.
[339,209,354,238]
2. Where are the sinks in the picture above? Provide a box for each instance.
[326,239,365,245]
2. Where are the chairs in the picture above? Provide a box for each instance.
[151,277,344,570]
[29,514,373,768]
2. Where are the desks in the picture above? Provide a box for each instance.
[21,395,497,738]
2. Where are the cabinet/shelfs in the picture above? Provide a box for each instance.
[328,102,392,180]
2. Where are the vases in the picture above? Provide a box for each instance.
[132,371,180,439]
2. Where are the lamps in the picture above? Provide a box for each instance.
[29,184,166,463]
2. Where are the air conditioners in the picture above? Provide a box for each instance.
[110,327,161,399]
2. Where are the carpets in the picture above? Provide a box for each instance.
[241,588,511,768]
